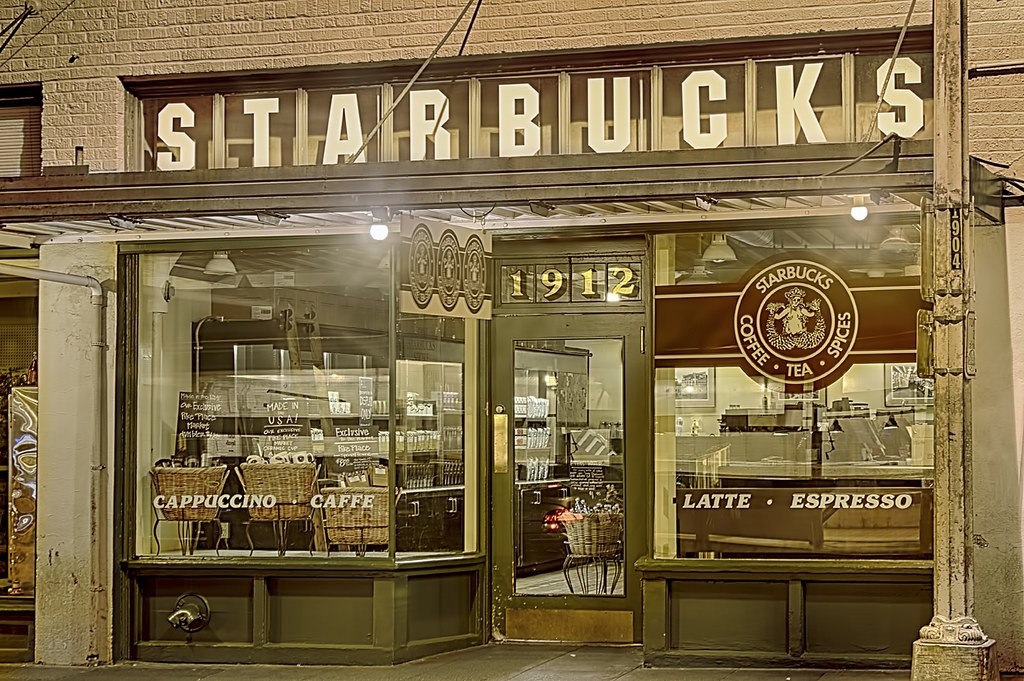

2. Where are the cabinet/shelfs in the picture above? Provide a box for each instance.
[232,343,465,552]
[517,370,571,577]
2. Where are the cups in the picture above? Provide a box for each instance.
[246,451,314,464]
[156,450,222,468]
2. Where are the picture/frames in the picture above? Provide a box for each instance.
[673,367,716,408]
[884,363,934,407]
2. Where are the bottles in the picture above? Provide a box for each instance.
[569,497,620,514]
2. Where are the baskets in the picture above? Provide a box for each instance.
[321,488,389,545]
[558,510,623,555]
[239,462,317,520]
[154,463,226,521]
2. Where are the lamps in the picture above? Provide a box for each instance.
[827,419,844,433]
[883,413,899,429]
[204,250,237,276]
[702,233,738,263]
